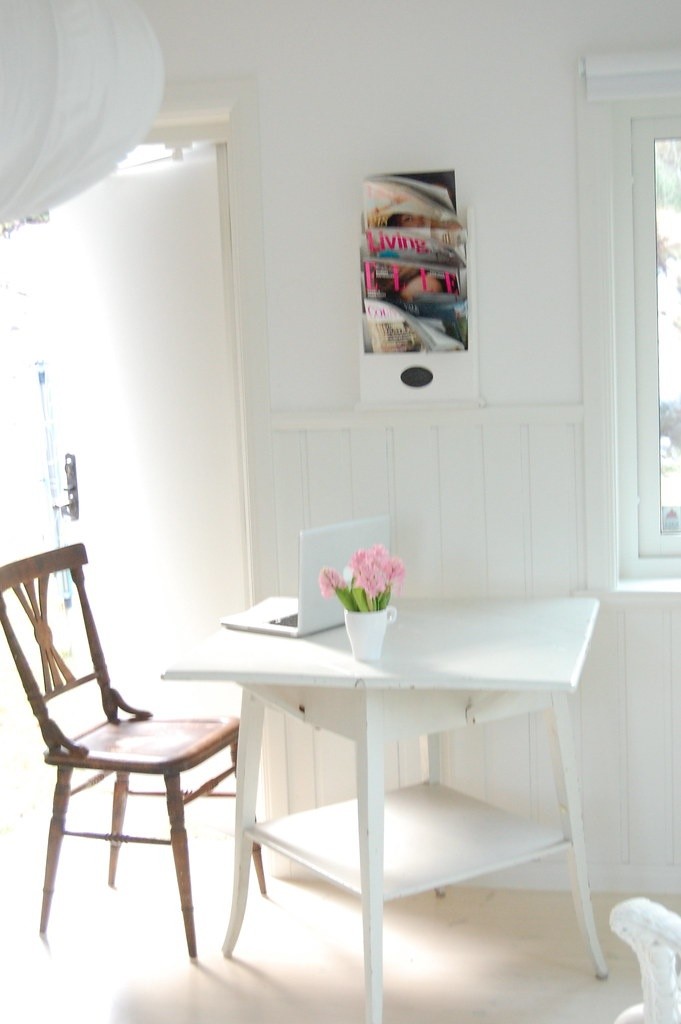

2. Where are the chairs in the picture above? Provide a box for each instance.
[0,543,266,957]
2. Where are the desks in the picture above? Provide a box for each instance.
[160,597,607,1024]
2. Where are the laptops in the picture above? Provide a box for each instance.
[220,515,389,638]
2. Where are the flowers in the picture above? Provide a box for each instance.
[319,543,406,611]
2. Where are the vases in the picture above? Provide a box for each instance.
[344,606,398,660]
